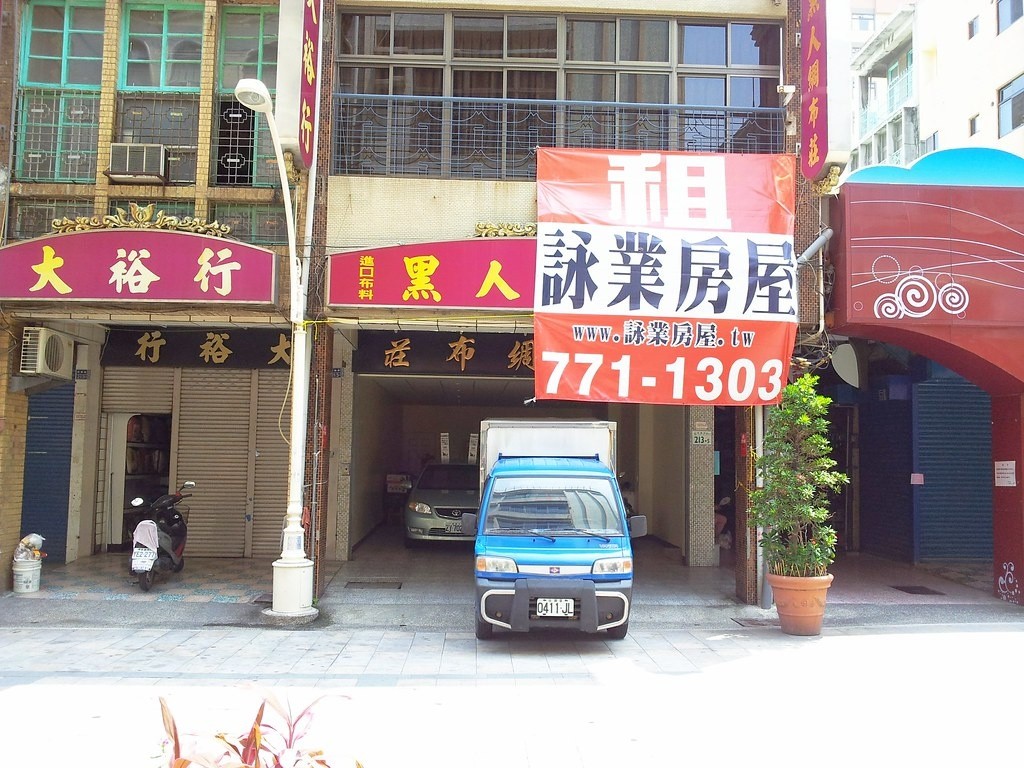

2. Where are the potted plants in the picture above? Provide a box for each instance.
[747,372,851,635]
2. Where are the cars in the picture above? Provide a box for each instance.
[400,459,480,549]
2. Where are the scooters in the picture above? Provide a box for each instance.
[129,481,197,591]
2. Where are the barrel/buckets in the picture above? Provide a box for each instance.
[12,560,41,592]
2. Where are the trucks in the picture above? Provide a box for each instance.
[461,415,649,639]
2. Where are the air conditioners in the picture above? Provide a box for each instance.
[20,326,74,383]
[108,141,169,184]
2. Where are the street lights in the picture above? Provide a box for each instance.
[234,78,315,614]
